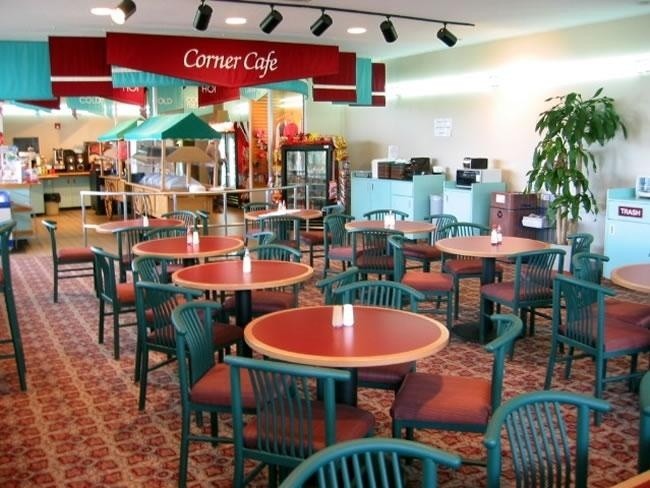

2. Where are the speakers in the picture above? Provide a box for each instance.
[76,153,85,172]
[63,150,76,172]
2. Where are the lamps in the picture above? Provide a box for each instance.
[192,0,213,31]
[260,0,457,47]
[109,0,137,25]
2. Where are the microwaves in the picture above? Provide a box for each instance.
[456,169,502,189]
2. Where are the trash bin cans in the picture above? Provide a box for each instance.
[44,193,60,216]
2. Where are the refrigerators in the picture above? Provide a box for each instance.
[280,144,338,229]
[204,121,249,208]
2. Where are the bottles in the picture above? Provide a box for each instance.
[383,209,397,230]
[241,247,253,272]
[330,303,354,329]
[143,214,149,227]
[186,228,201,248]
[491,223,502,244]
[278,199,287,213]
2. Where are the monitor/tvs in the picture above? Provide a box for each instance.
[13,137,39,154]
